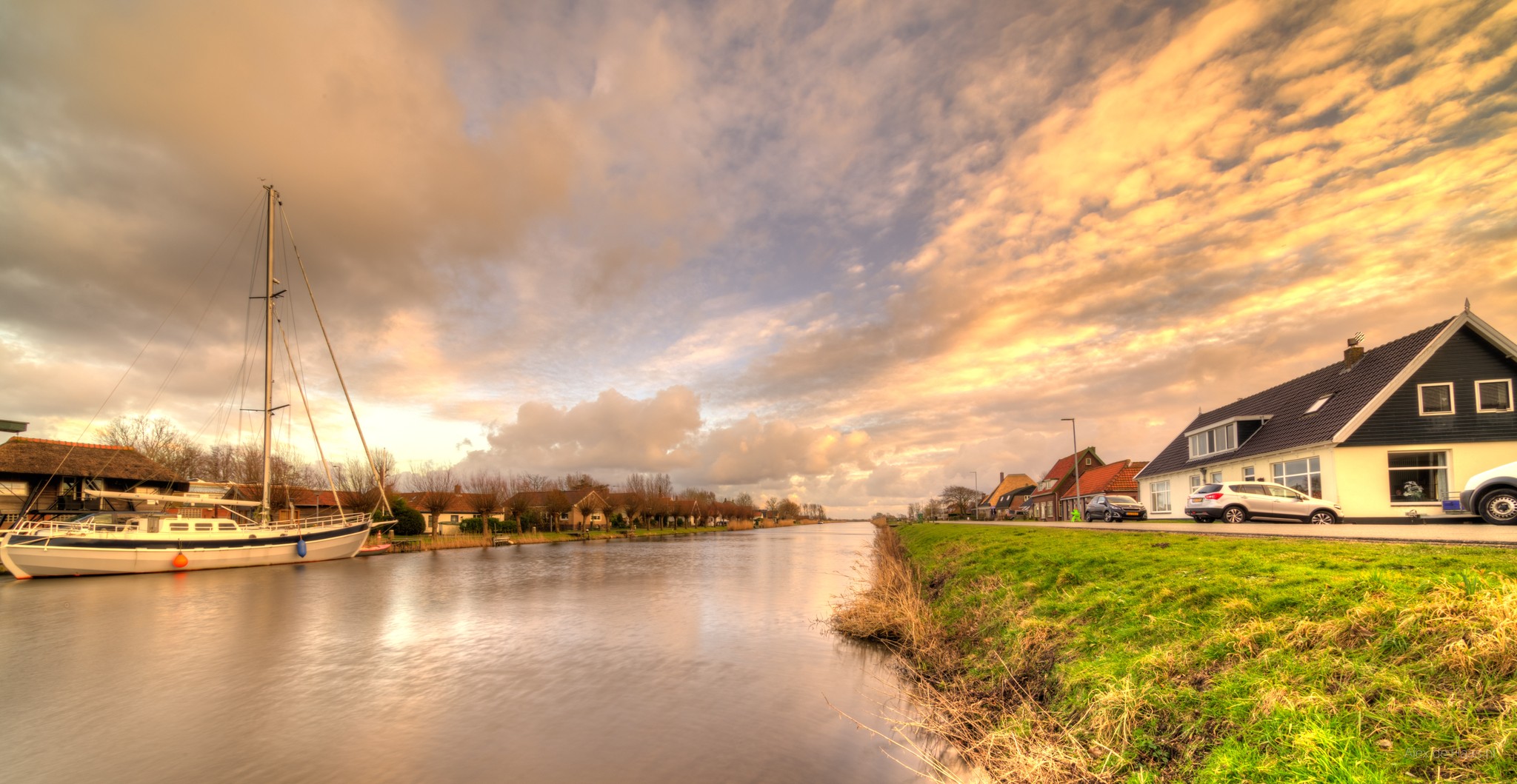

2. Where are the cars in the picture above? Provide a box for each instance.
[1086,494,1147,522]
[1185,480,1344,527]
[1459,462,1517,525]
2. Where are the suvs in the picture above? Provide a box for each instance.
[1005,509,1030,521]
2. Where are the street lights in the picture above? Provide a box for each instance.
[929,495,933,520]
[1061,418,1082,518]
[940,490,944,520]
[970,471,978,520]
[920,500,925,519]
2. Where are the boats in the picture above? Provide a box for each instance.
[819,520,823,524]
[357,544,392,557]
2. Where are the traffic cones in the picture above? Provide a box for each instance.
[376,529,382,541]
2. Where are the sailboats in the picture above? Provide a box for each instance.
[0,182,397,580]
